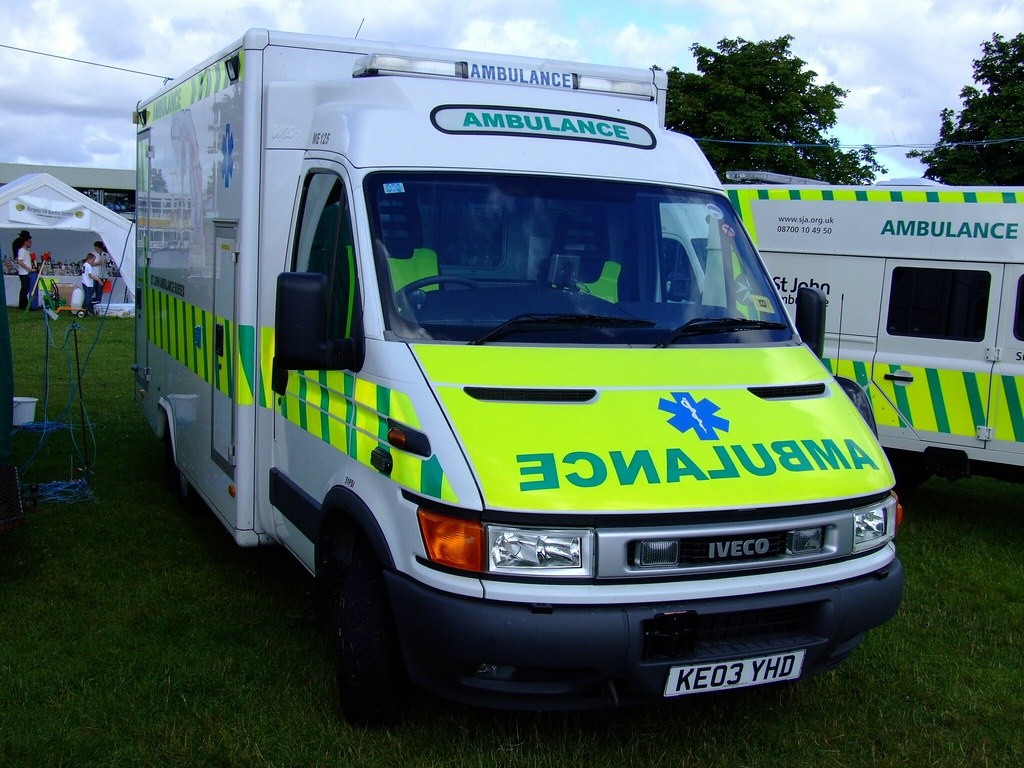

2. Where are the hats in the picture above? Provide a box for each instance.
[17,230,29,236]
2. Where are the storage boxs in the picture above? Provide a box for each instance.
[54,283,74,306]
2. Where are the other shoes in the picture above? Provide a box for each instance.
[19,305,35,311]
[87,310,93,316]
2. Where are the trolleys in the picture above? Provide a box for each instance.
[49,278,87,318]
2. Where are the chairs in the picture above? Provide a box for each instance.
[542,211,621,304]
[331,188,444,340]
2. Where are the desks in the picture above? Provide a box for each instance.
[3,274,127,307]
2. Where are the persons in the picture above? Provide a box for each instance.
[81,242,109,316]
[12,230,36,310]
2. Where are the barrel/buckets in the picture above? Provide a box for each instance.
[71,289,83,308]
[13,397,38,426]
[168,394,198,423]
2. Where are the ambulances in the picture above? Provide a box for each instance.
[655,168,1023,490]
[132,25,904,734]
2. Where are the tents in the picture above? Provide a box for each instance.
[0,174,135,307]
